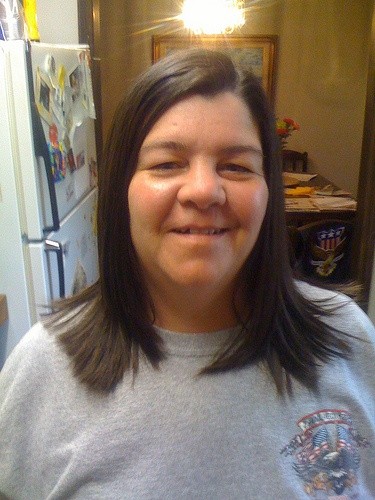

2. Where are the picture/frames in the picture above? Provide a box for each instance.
[152,33,277,110]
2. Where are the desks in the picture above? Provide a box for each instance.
[277,177,359,285]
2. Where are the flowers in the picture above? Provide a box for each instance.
[276,118,299,146]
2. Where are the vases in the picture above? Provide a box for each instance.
[280,149,301,174]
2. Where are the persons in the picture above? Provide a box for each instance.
[0,48,375,500]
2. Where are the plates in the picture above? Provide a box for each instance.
[282,175,299,186]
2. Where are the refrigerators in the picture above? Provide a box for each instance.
[0,40,99,367]
